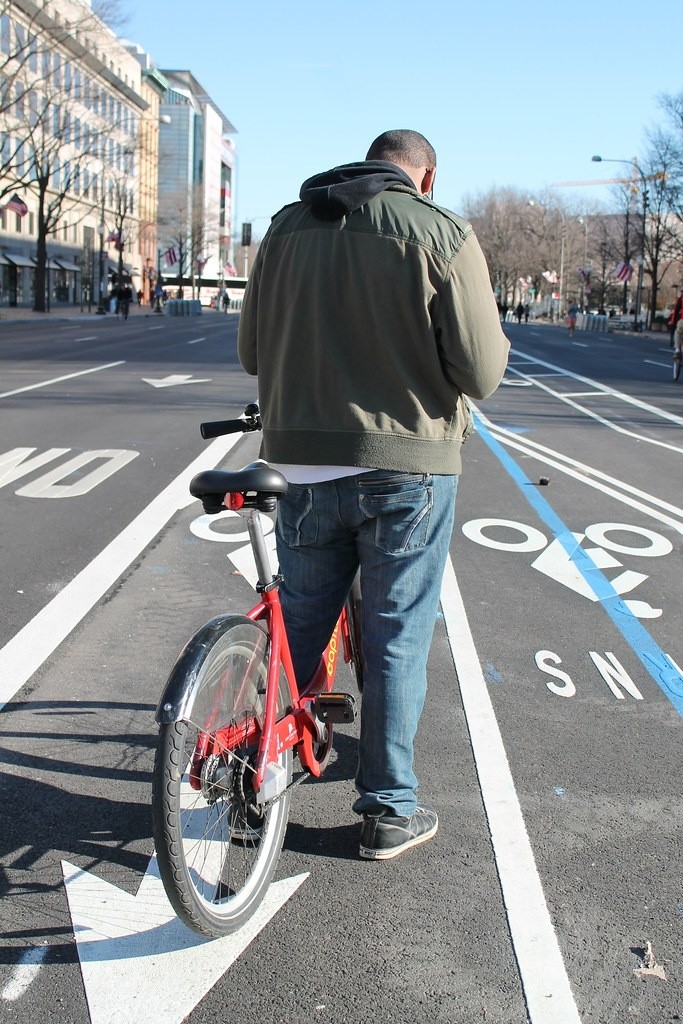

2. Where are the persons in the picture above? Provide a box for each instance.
[497,291,683,363]
[109,282,230,318]
[235,129,511,862]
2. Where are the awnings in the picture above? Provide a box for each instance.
[0,254,138,277]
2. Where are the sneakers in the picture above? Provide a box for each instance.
[358,803,439,860]
[227,799,263,841]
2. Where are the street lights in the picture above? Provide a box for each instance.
[95,114,172,315]
[527,199,564,321]
[577,218,589,317]
[591,156,648,328]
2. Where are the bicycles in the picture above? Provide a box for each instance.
[150,404,361,940]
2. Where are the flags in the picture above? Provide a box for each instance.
[166,246,180,266]
[110,230,125,251]
[5,195,28,215]
[521,258,633,291]
[195,258,236,278]
